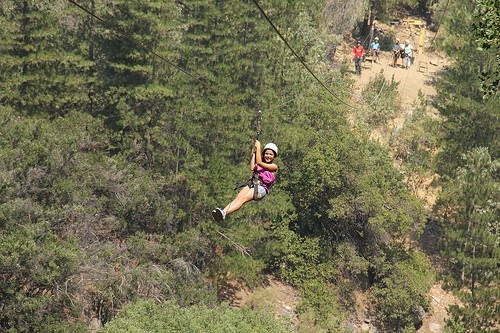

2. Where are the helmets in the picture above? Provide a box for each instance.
[357,41,361,44]
[262,143,278,158]
[375,36,379,40]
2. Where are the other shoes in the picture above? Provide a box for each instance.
[212,210,220,223]
[216,207,225,220]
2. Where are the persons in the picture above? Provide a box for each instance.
[212,140,278,223]
[370,37,381,64]
[402,39,413,69]
[391,38,401,68]
[353,41,364,76]
